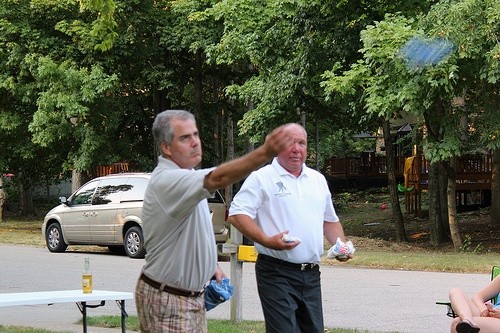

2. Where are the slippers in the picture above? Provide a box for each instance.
[455,319,481,333]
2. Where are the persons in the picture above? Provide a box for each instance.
[134,110,294,333]
[449,274,500,333]
[228,122,352,333]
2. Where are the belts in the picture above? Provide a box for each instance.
[257,253,314,272]
[140,272,217,298]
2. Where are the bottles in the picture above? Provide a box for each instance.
[82,258,92,295]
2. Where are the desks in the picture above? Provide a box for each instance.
[0,290,134,333]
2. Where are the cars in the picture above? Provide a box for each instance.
[41,172,230,260]
